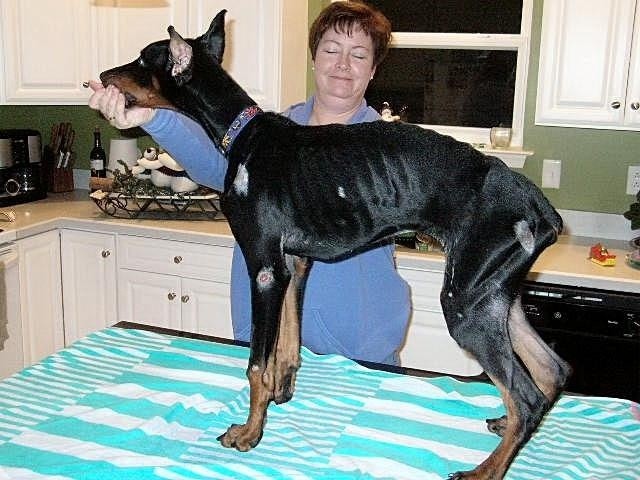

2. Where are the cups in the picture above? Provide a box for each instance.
[490,126,511,148]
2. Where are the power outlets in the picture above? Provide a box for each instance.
[626,163,640,196]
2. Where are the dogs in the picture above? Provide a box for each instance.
[98,9,575,480]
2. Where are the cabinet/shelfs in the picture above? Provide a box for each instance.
[2,0,186,112]
[394,268,487,378]
[59,231,118,357]
[117,235,235,344]
[190,2,282,111]
[0,234,64,380]
[532,0,640,132]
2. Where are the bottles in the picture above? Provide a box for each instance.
[88,126,107,180]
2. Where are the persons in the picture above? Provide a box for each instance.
[86,0,412,367]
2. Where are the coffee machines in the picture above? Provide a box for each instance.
[1,129,46,209]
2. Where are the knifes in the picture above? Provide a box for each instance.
[51,119,75,175]
